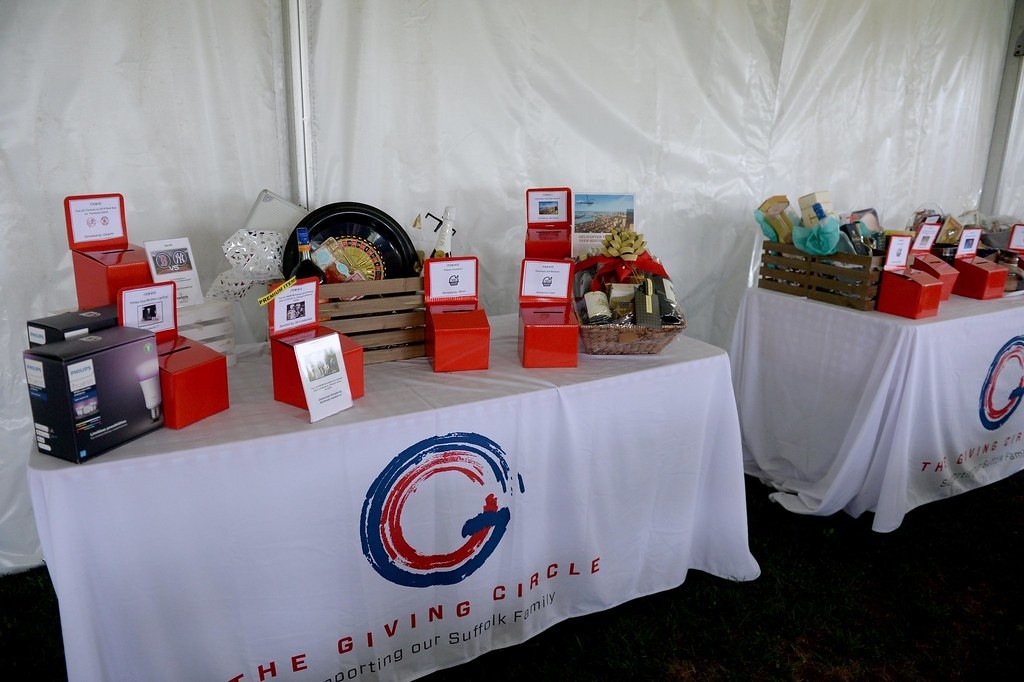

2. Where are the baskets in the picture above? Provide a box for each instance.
[571,289,687,354]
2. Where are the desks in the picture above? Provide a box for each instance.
[25,309,760,682]
[724,283,1024,534]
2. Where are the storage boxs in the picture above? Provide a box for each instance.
[21,326,168,467]
[759,240,885,312]
[28,303,119,350]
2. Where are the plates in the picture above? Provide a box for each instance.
[282,201,420,337]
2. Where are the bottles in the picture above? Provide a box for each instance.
[576,254,613,325]
[291,227,330,322]
[410,207,455,327]
[811,203,874,300]
